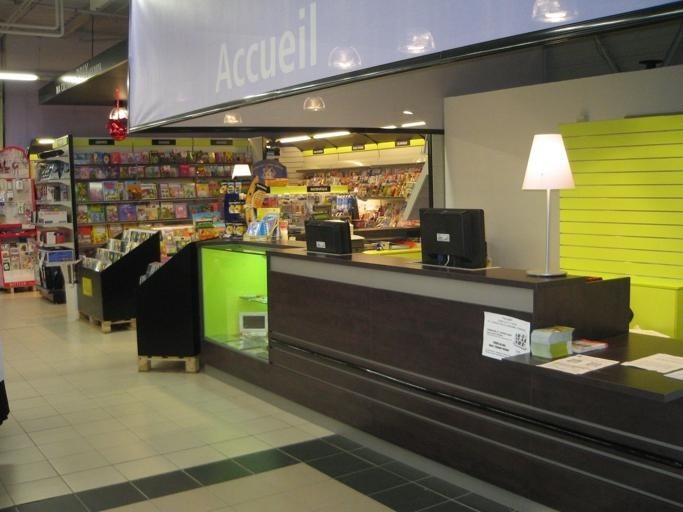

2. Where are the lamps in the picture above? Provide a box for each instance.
[521,132,577,275]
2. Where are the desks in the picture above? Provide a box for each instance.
[267,245,681,510]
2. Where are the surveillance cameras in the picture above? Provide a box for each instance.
[265,147,281,156]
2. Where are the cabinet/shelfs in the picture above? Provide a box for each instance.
[28,124,426,374]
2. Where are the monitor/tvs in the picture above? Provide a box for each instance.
[239,312,268,337]
[418,208,487,270]
[304,219,352,254]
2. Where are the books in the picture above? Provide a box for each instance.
[530,325,609,359]
[29,148,253,286]
